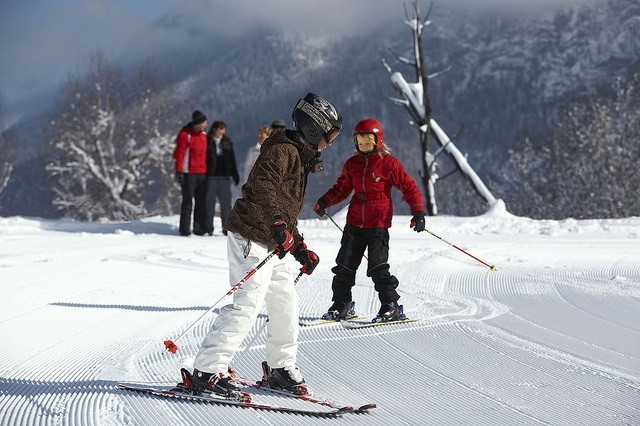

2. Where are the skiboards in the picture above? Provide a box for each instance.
[117,378,376,415]
[298,316,421,329]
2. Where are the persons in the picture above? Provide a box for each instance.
[207,121,240,235]
[313,117,426,322]
[174,110,208,236]
[242,125,271,186]
[270,119,284,136]
[192,92,342,395]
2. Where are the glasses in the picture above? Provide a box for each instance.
[325,128,341,144]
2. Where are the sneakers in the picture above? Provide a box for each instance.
[193,369,241,399]
[372,301,403,323]
[269,368,308,395]
[323,299,356,319]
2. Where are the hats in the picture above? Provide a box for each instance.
[192,110,207,124]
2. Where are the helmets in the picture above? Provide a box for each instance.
[353,117,384,151]
[292,92,343,146]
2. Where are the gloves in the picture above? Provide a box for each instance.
[314,199,326,216]
[270,221,294,259]
[410,212,426,232]
[290,240,320,275]
[176,173,185,184]
